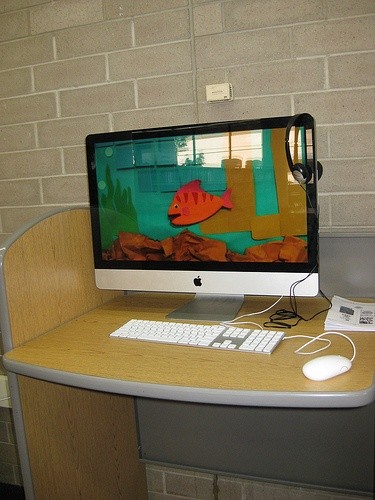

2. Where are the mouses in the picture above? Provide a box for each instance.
[301,355,351,381]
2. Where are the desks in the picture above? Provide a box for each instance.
[0,205,375,500]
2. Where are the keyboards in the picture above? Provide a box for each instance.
[109,318,285,355]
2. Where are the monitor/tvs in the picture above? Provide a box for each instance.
[84,114,320,321]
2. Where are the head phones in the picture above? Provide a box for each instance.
[284,113,323,184]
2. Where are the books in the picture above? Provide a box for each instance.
[324,296,375,332]
